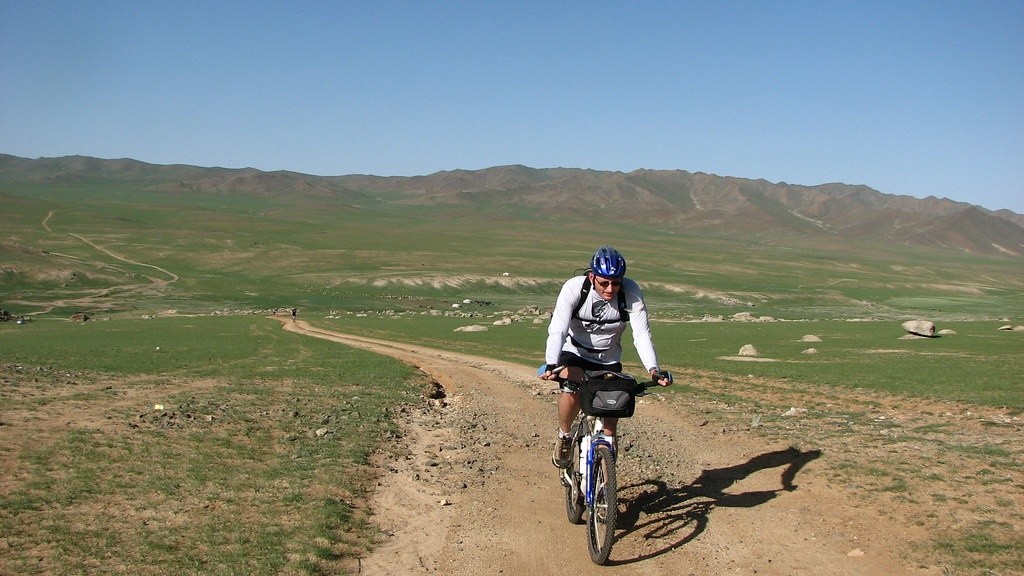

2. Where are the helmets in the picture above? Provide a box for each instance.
[590,247,626,279]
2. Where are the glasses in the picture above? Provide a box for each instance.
[596,278,622,287]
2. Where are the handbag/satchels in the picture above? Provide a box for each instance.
[579,370,636,418]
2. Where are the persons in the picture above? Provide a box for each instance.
[272,307,277,315]
[291,308,297,323]
[537,245,673,486]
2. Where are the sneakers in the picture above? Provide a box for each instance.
[552,437,574,469]
[599,441,618,466]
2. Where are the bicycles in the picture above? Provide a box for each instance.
[542,368,659,564]
